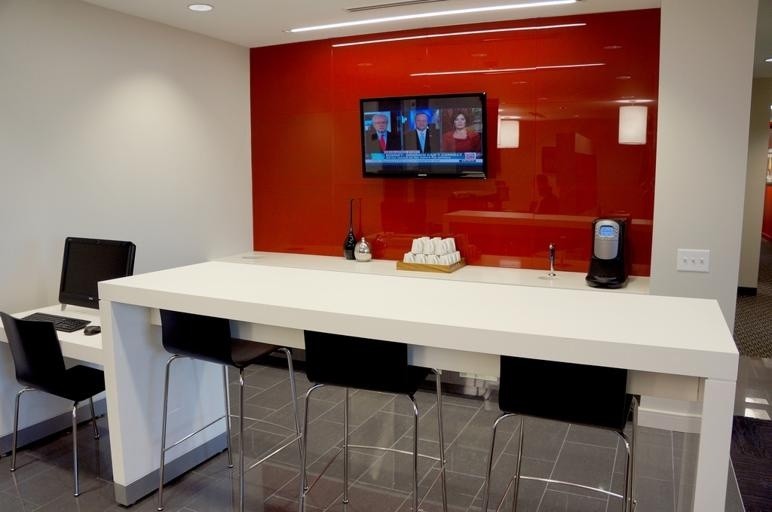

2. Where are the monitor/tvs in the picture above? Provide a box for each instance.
[58,237,135,310]
[360,92,487,180]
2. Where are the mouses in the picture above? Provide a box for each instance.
[85,325,100,335]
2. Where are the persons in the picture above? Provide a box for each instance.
[404,113,439,153]
[367,114,400,156]
[443,113,480,152]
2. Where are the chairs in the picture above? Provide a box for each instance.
[0,311,106,497]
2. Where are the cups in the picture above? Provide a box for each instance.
[403,236,461,266]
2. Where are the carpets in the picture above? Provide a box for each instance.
[732,242,772,359]
[729,415,772,512]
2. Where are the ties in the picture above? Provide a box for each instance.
[379,135,386,152]
[419,132,426,153]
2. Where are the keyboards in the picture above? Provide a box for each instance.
[21,313,91,332]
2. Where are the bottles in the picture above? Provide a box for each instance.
[344,198,357,260]
[354,237,372,262]
